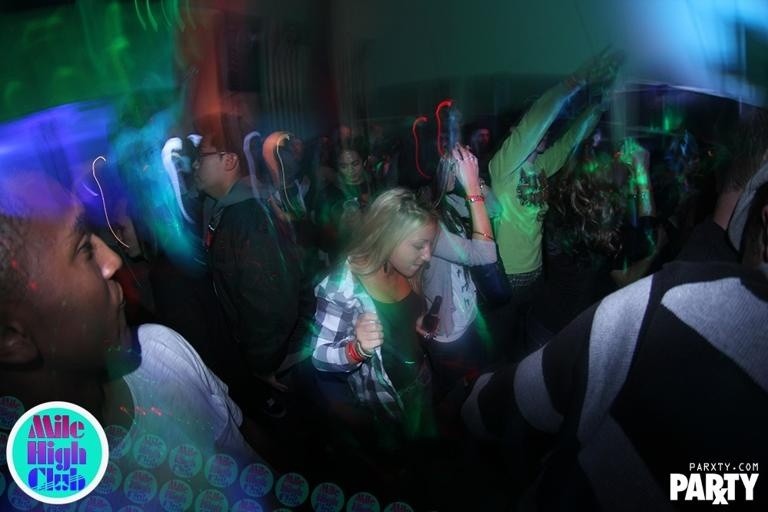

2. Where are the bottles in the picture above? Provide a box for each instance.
[417,295,441,355]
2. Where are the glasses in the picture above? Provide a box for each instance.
[196,150,225,161]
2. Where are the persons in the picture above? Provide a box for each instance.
[0,56,767,511]
